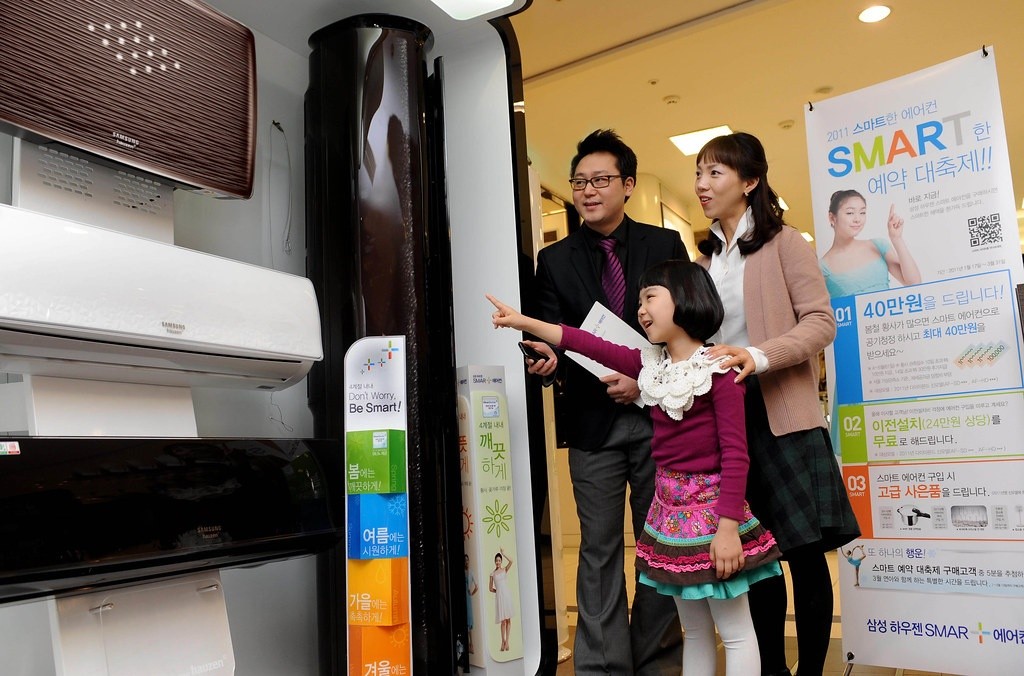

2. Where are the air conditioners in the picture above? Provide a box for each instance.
[0,201,324,393]
[0,434,345,606]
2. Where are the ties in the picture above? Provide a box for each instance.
[599,238,626,321]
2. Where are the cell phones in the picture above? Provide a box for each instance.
[518,342,550,363]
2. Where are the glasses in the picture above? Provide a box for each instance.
[569,175,621,191]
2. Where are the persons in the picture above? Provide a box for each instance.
[485,127,861,676]
[819,189,921,299]
